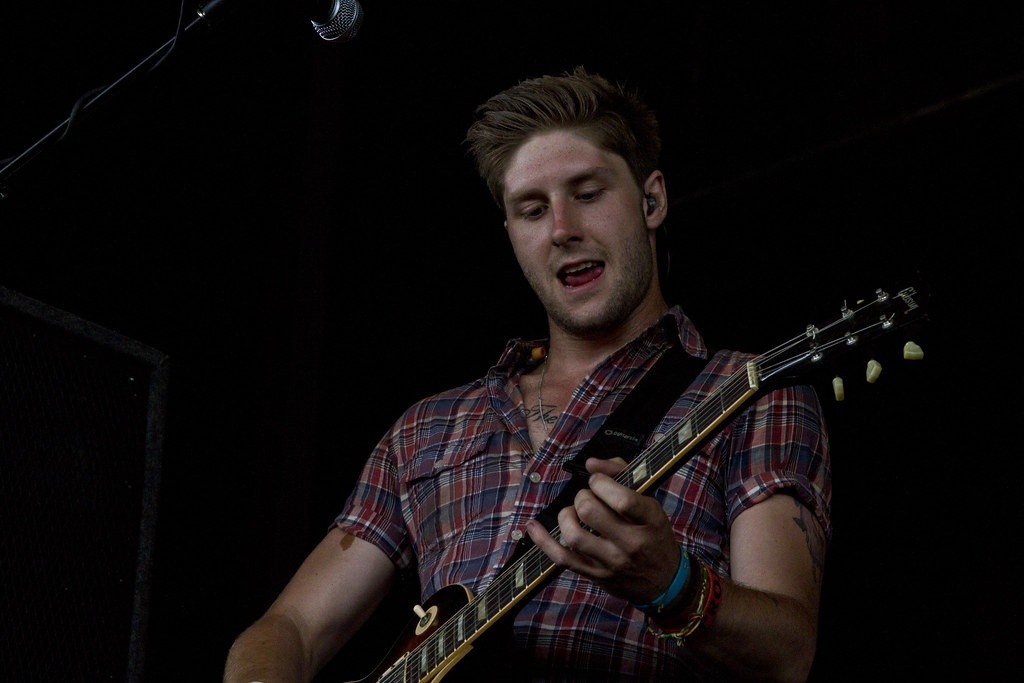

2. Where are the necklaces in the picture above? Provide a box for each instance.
[539,357,549,438]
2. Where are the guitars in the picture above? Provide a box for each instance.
[323,267,937,683]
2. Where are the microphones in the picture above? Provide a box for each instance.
[292,0,365,45]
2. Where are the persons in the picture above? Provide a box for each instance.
[222,63,835,683]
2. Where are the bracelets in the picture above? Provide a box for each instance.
[645,560,722,648]
[628,544,690,614]
